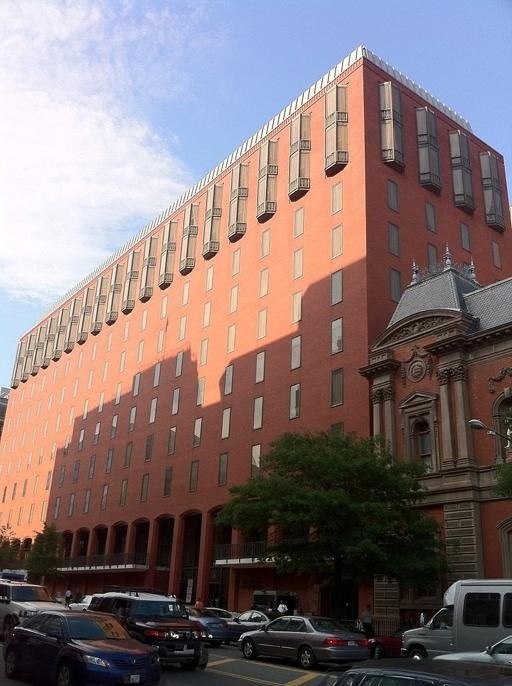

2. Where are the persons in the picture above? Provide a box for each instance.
[50,588,83,608]
[195,598,204,608]
[277,599,287,614]
[361,604,375,637]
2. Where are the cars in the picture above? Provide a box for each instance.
[187,605,414,670]
[1,608,161,686]
[68,593,92,610]
[432,630,512,662]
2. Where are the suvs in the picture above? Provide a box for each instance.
[0,578,70,642]
[87,591,215,672]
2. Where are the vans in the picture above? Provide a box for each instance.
[400,578,512,659]
